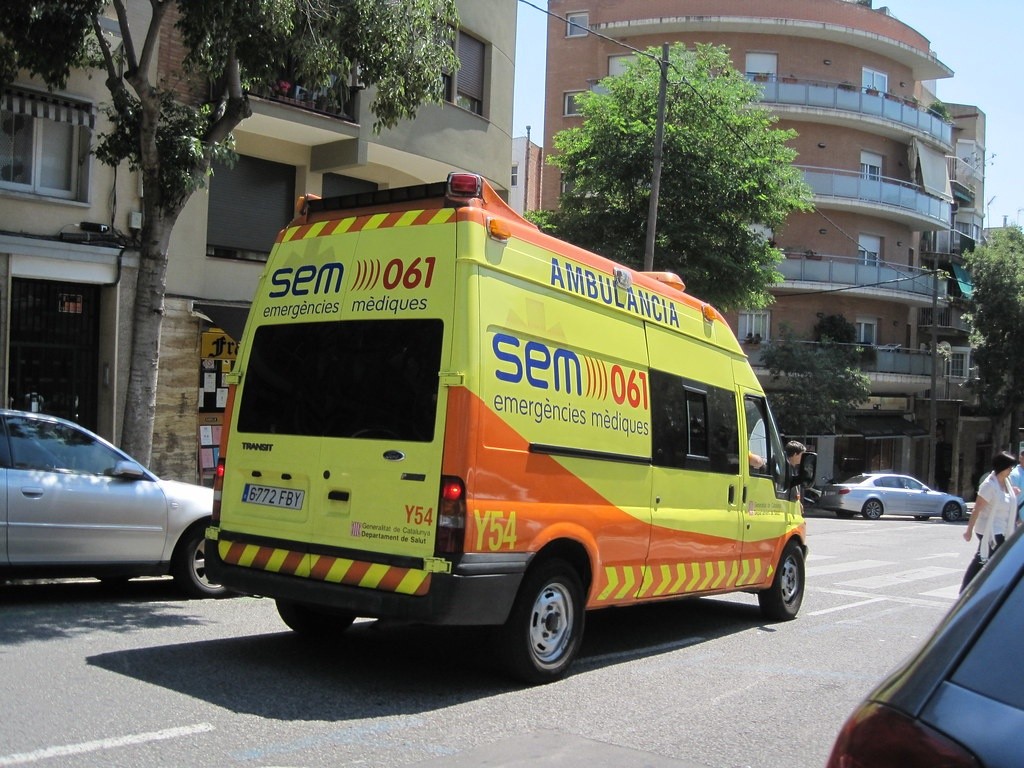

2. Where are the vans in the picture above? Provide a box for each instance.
[204,171,819,679]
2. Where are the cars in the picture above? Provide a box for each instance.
[823,497,1024,768]
[1,409,231,597]
[819,472,967,522]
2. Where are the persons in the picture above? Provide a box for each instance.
[786,441,806,516]
[1009,452,1024,523]
[960,451,1021,593]
